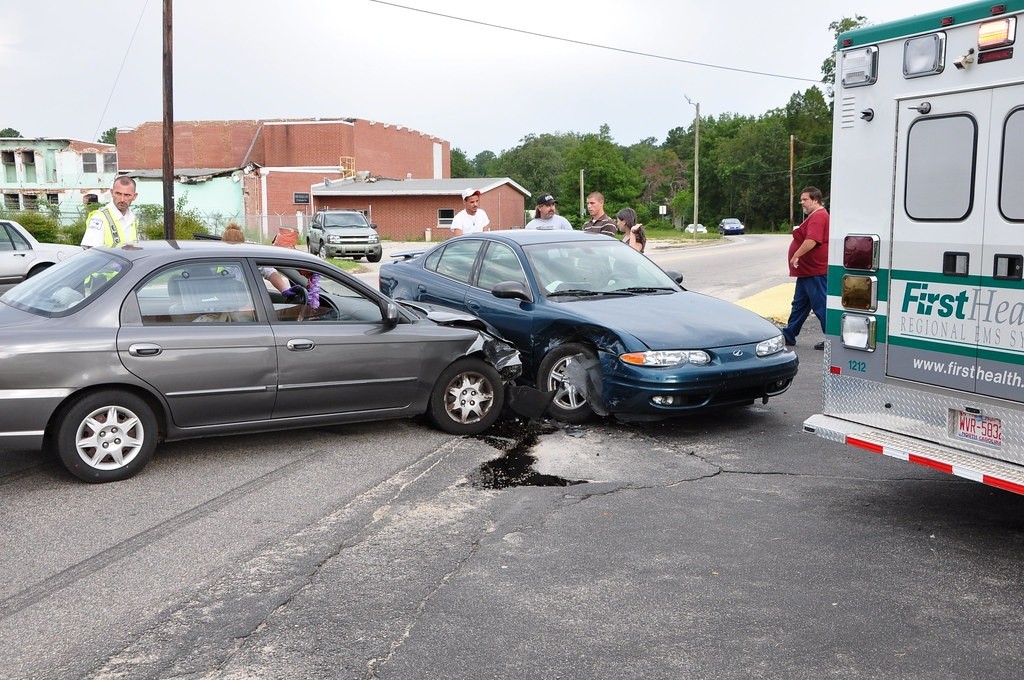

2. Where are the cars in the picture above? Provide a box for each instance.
[0,240,523,483]
[0,219,85,281]
[379,229,800,424]
[718,217,745,236]
[684,224,708,233]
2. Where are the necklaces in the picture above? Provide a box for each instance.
[471,219,476,226]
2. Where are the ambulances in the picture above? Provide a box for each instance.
[802,0,1024,497]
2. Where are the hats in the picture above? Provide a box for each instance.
[537,194,558,205]
[461,188,481,200]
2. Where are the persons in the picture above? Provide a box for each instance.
[80,176,138,298]
[613,208,646,272]
[780,187,830,350]
[450,188,489,236]
[573,191,616,270]
[525,193,573,258]
[222,222,302,296]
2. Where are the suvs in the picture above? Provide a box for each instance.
[306,208,383,262]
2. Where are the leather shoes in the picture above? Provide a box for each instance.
[814,341,824,350]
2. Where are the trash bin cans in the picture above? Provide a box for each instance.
[424,228,432,242]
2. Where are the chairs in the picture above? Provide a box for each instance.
[168,273,254,323]
